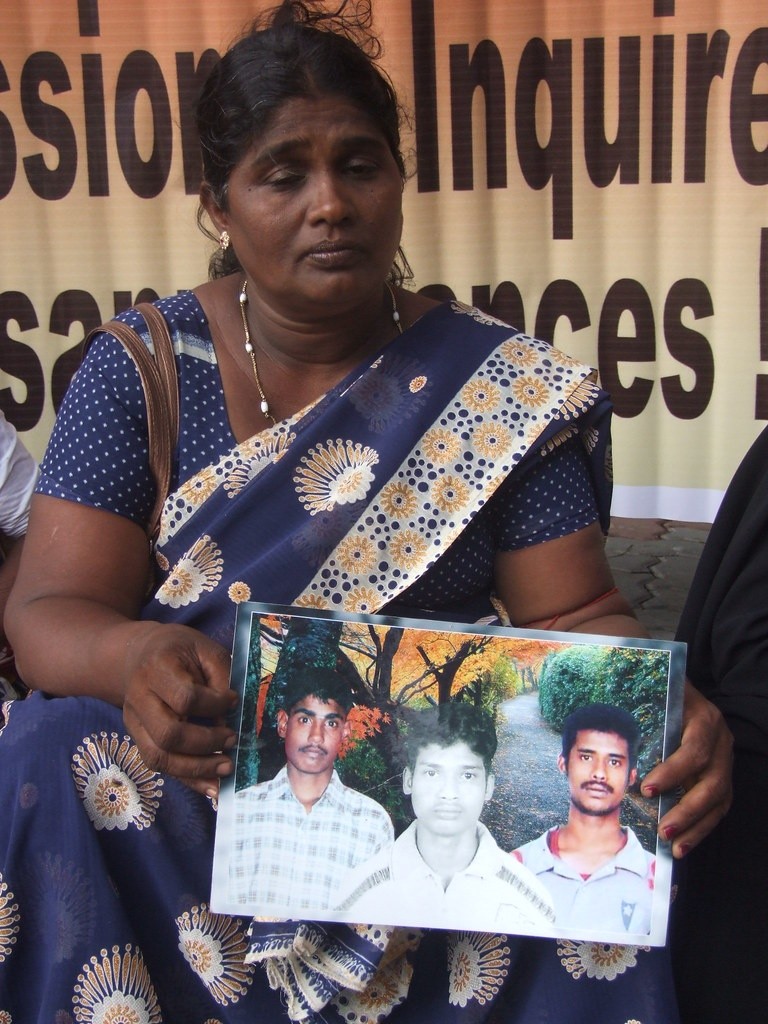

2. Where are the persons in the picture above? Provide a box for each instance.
[328,698,561,938]
[505,701,673,947]
[211,668,394,950]
[0,0,768,1024]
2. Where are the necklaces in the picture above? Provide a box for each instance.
[239,279,406,424]
